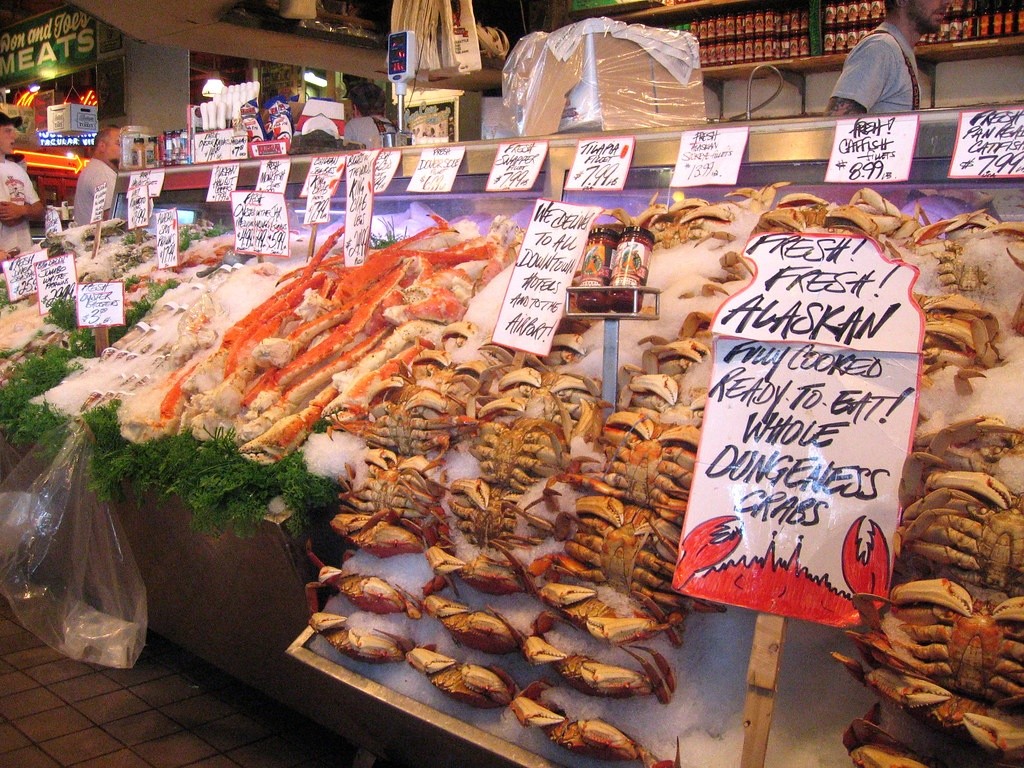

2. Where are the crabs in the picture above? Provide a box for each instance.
[154,186,1024,768]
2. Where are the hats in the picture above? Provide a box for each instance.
[0,112,22,128]
[343,82,385,108]
[5,150,25,163]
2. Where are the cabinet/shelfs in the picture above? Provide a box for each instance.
[562,0,1024,83]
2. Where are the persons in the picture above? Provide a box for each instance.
[73,128,121,226]
[824,0,951,116]
[345,83,397,149]
[0,112,46,268]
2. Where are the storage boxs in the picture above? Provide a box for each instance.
[47,102,98,137]
[500,16,709,138]
[193,98,345,166]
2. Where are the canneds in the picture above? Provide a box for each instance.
[691,0,978,69]
[576,227,619,312]
[605,226,655,313]
